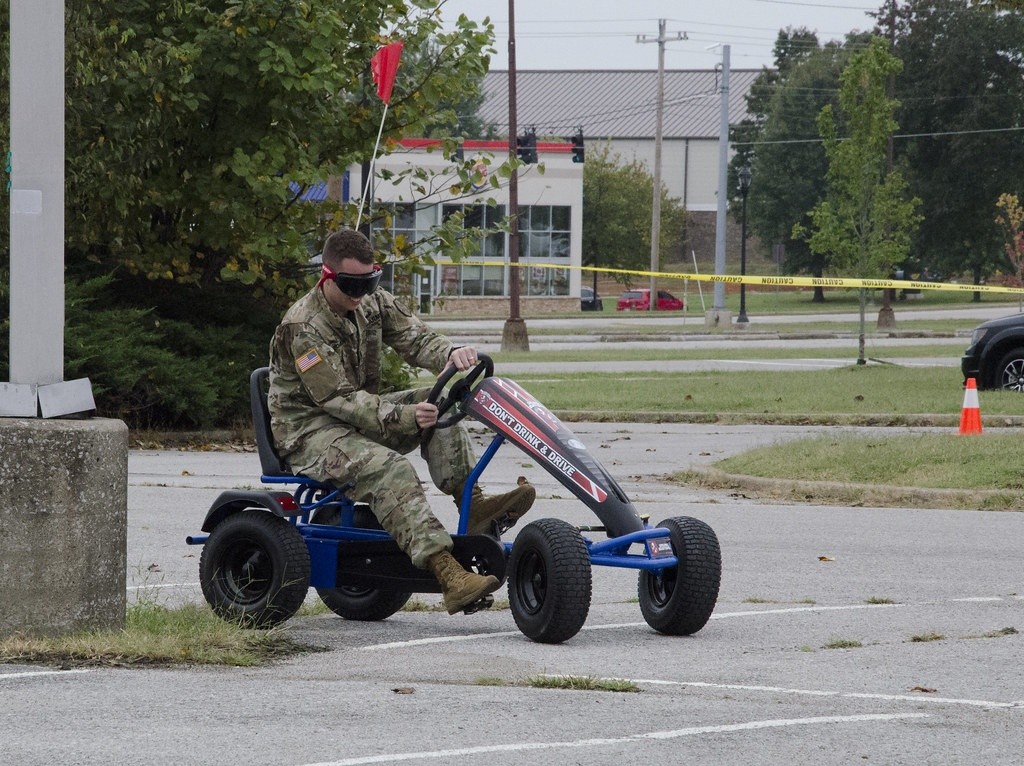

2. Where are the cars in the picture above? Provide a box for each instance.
[581,286,603,311]
[616,289,689,312]
[961,312,1024,393]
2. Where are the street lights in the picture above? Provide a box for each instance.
[877,1,907,330]
[591,238,600,311]
[737,165,753,323]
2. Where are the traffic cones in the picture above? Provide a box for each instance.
[951,378,986,436]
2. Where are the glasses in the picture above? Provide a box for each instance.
[317,260,382,297]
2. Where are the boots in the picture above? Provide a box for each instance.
[431,551,499,615]
[453,482,536,534]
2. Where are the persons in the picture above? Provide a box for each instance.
[267,230,536,616]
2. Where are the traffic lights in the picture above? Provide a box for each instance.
[571,134,585,163]
[517,133,538,165]
